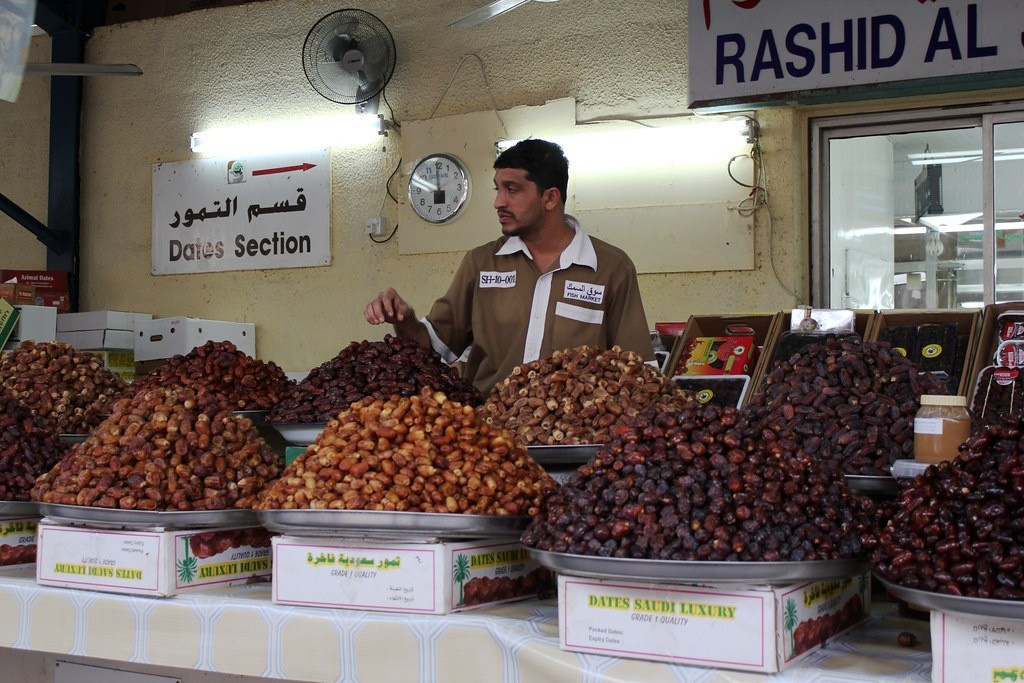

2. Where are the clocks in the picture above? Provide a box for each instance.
[408,153,473,225]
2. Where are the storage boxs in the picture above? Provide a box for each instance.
[3,305,57,350]
[556,570,873,673]
[0,296,20,354]
[930,609,1024,683]
[55,329,135,350]
[0,283,36,306]
[270,535,558,615]
[133,316,256,362]
[81,350,134,381]
[0,269,70,311]
[35,522,280,598]
[0,515,44,570]
[56,310,153,331]
[650,301,1024,411]
[894,223,958,262]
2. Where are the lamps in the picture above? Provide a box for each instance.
[894,226,931,235]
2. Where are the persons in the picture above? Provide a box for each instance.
[363,139,662,403]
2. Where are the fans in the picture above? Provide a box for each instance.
[301,7,397,115]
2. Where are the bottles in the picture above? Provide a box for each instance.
[913,394,972,464]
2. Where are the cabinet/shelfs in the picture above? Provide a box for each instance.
[894,225,966,309]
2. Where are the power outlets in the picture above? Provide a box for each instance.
[367,217,386,236]
